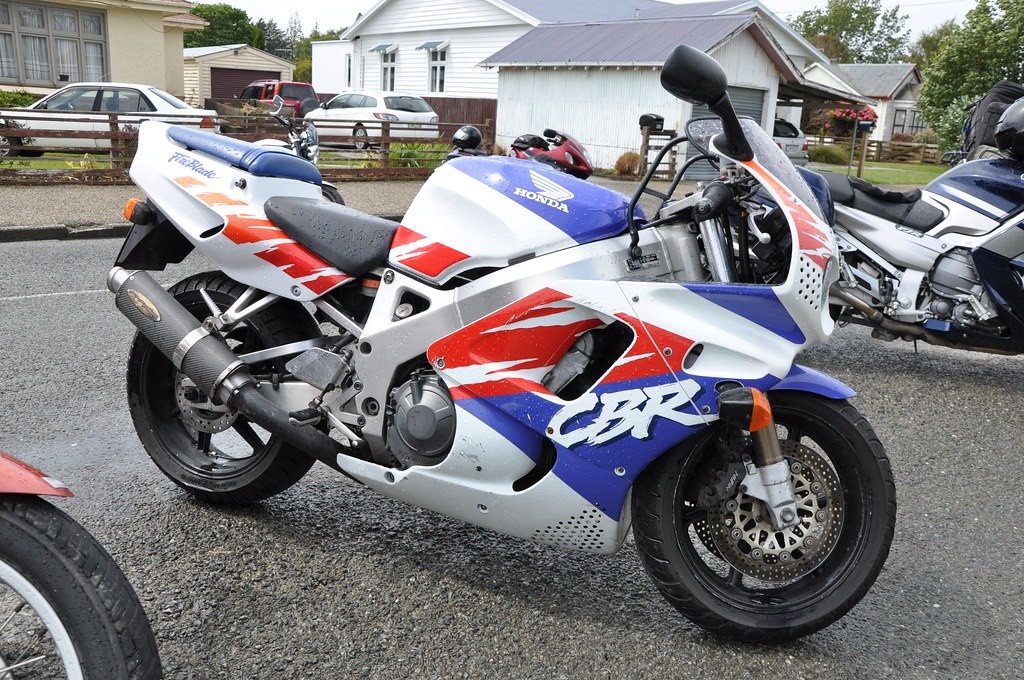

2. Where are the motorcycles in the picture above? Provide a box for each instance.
[442,125,595,179]
[107,43,897,646]
[811,158,1024,355]
[0,451,162,679]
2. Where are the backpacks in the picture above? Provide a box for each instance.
[962,80,1024,153]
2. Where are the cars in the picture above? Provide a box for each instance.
[772,118,810,168]
[302,91,439,149]
[0,82,220,157]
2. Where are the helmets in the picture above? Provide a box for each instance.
[994,97,1024,151]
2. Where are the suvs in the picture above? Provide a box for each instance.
[233,79,320,121]
